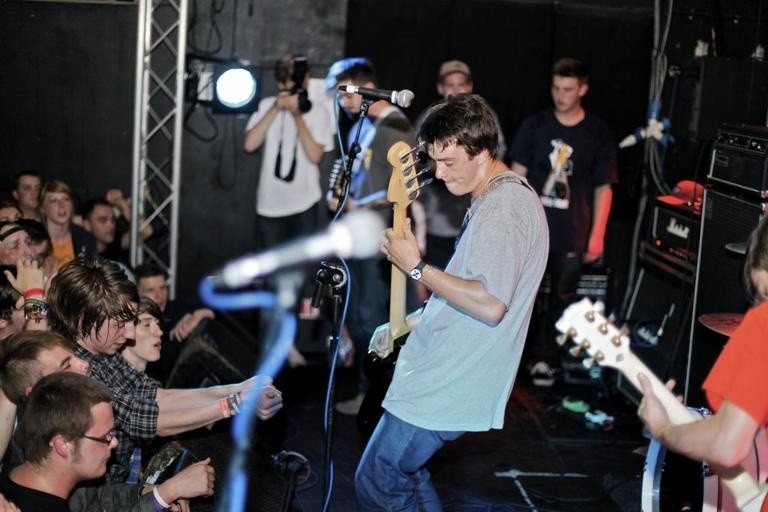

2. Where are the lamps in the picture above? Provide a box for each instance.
[209,53,264,117]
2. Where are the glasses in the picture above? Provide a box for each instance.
[83,428,118,444]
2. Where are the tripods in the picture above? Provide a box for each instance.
[312,98,378,512]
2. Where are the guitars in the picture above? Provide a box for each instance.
[548,291,733,512]
[352,136,434,444]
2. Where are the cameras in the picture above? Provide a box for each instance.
[278,56,312,113]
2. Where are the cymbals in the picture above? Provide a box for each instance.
[697,311,745,338]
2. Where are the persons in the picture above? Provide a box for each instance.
[1,372,117,512]
[354,92,548,512]
[413,61,505,276]
[39,180,96,262]
[0,194,23,225]
[246,54,334,249]
[637,213,768,512]
[0,329,214,512]
[0,215,46,263]
[134,262,213,360]
[511,61,615,386]
[121,296,163,372]
[0,254,50,335]
[47,258,282,481]
[14,173,40,217]
[324,55,426,412]
[80,188,152,259]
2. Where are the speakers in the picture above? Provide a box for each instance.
[692,121,768,377]
[616,264,695,404]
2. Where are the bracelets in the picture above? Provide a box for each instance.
[21,288,45,298]
[409,260,427,282]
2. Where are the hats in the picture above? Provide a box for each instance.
[658,181,705,207]
[441,61,472,79]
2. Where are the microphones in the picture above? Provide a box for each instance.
[338,84,415,107]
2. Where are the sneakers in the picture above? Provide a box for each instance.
[533,361,555,386]
